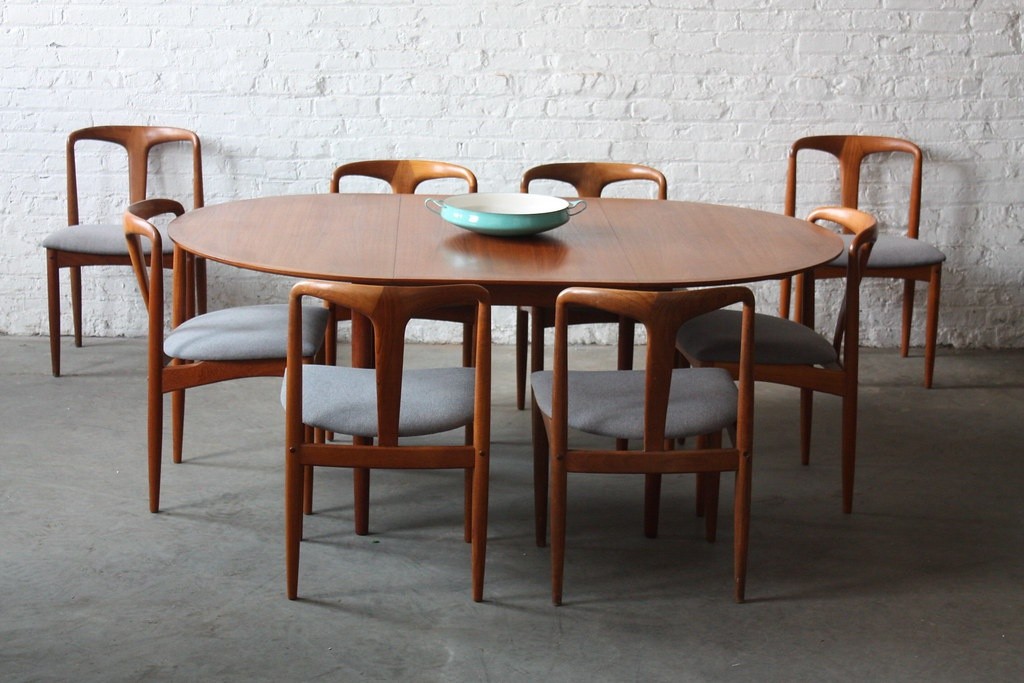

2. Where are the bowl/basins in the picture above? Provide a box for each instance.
[424,193,587,237]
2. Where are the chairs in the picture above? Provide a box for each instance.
[40,125,209,378]
[278,279,492,605]
[515,161,667,410]
[529,285,756,607]
[324,158,478,443]
[662,207,879,519]
[121,198,335,516]
[779,133,948,391]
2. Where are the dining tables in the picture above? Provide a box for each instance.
[167,193,846,541]
